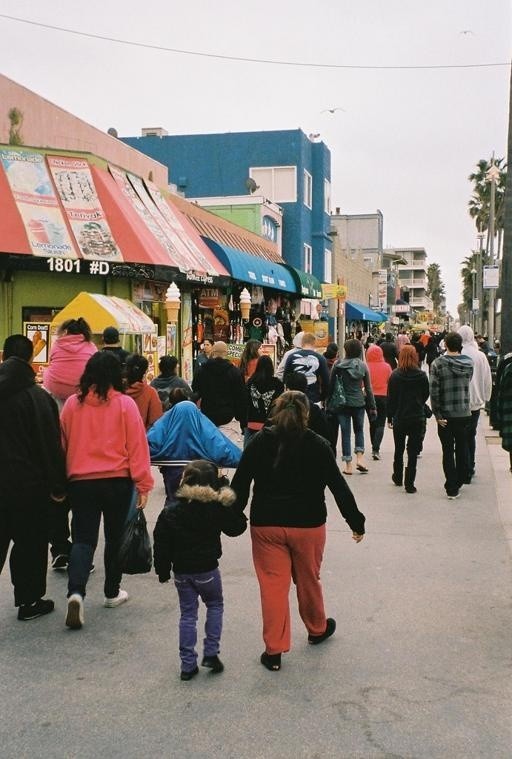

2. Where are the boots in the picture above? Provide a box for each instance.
[405,467,417,492]
[392,462,403,486]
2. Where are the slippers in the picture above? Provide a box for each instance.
[342,455,379,474]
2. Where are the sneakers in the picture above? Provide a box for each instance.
[201,655,223,672]
[261,652,281,671]
[17,555,129,628]
[181,666,199,681]
[308,618,336,644]
[447,483,463,498]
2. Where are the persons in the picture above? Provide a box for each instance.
[57,349,156,628]
[43,318,100,575]
[0,333,61,621]
[230,390,366,672]
[151,460,248,682]
[95,317,502,510]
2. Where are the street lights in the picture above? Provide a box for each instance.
[368,293,372,335]
[483,150,502,349]
[467,229,485,339]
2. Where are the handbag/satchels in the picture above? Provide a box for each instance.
[326,375,346,411]
